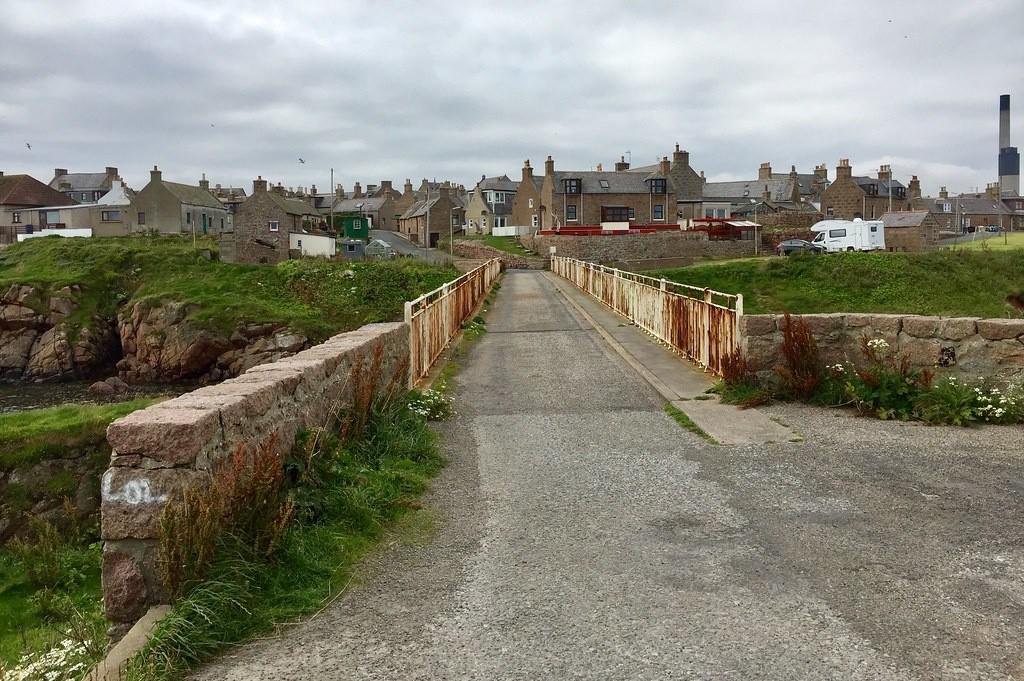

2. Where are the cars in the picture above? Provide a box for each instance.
[775,239,822,256]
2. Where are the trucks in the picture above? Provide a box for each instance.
[810,217,886,253]
